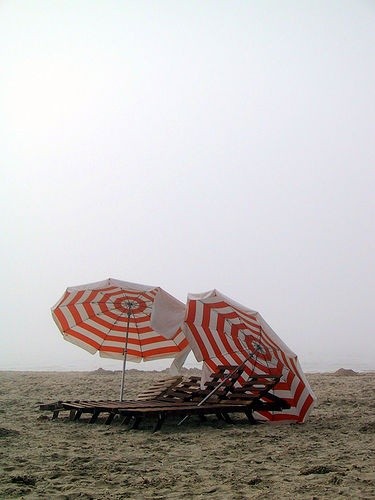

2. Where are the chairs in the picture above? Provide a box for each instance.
[95,364,246,426]
[39,374,184,421]
[118,373,291,435]
[61,376,202,425]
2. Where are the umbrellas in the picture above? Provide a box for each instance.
[177,287,317,426]
[50,278,191,402]
